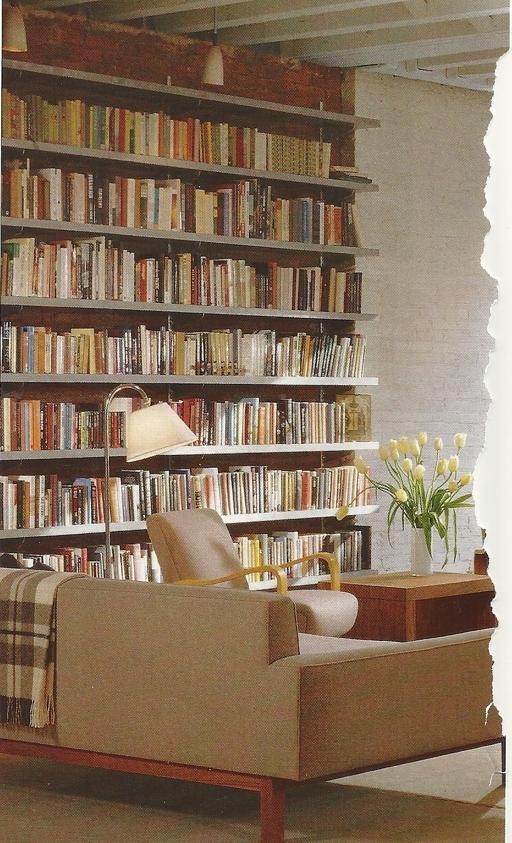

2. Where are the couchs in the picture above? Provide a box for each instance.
[0,567,505,842]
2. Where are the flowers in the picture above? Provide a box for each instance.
[336,431,474,570]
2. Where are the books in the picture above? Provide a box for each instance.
[1,83,373,591]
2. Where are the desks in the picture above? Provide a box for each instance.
[318,570,497,642]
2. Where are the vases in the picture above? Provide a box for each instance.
[409,528,433,577]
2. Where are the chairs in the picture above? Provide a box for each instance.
[146,509,358,636]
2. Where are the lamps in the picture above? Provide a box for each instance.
[2,0,29,51]
[103,383,198,580]
[201,9,225,85]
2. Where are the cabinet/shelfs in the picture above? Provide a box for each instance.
[0,59,382,542]
